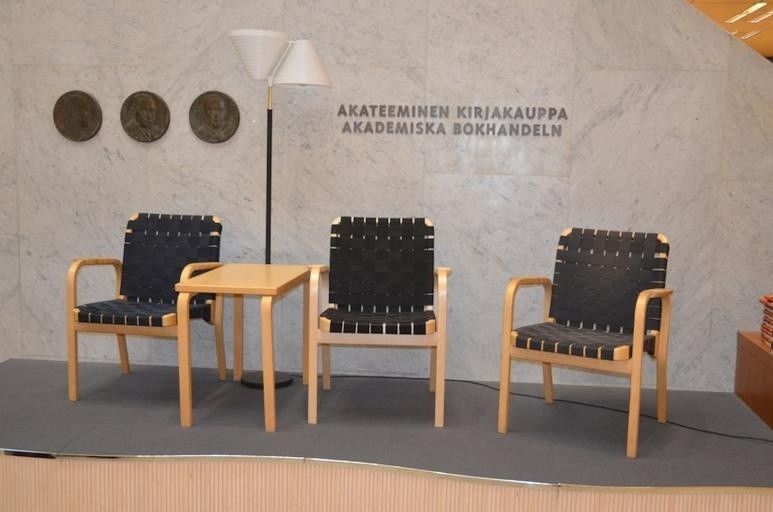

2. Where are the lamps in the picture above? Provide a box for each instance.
[228,29,332,390]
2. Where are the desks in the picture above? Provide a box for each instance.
[174,263,314,433]
[734,329,773,431]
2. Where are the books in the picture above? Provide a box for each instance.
[756,292,772,350]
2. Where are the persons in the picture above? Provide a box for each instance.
[58,95,101,142]
[125,94,163,142]
[192,95,235,142]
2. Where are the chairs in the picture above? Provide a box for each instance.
[66,213,227,428]
[498,225,675,458]
[308,216,451,427]
